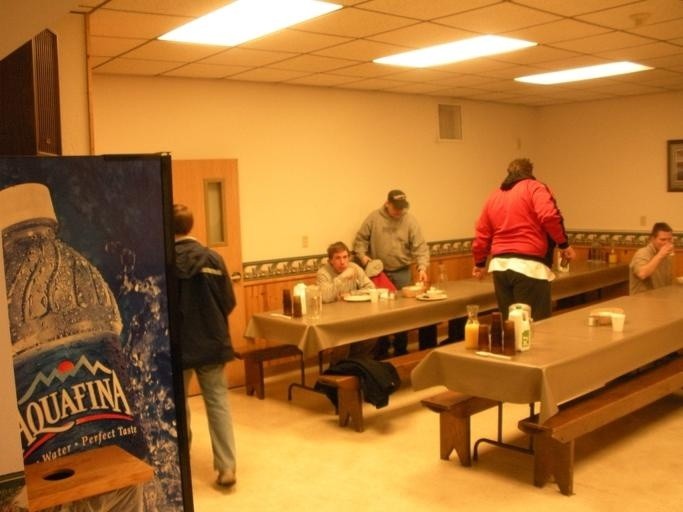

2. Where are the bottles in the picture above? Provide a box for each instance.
[490,312,502,353]
[477,323,486,350]
[282,287,292,316]
[0,182,169,511]
[292,294,301,318]
[586,241,617,264]
[463,304,478,349]
[503,319,515,356]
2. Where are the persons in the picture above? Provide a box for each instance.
[316,242,375,367]
[169,205,237,488]
[472,158,577,324]
[628,221,674,296]
[352,189,429,359]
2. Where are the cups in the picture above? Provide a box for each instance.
[611,313,625,332]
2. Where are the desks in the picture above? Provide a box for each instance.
[414,280,683,472]
[247,251,634,410]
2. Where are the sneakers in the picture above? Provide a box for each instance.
[217,469,236,486]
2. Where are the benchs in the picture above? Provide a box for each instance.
[319,345,433,432]
[419,388,508,466]
[518,356,682,497]
[232,339,305,400]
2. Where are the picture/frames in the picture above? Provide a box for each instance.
[664,139,682,193]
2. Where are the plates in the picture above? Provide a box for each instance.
[343,295,370,302]
[415,293,447,300]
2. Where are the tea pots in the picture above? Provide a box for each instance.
[304,285,323,322]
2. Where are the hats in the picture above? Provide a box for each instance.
[389,190,409,208]
